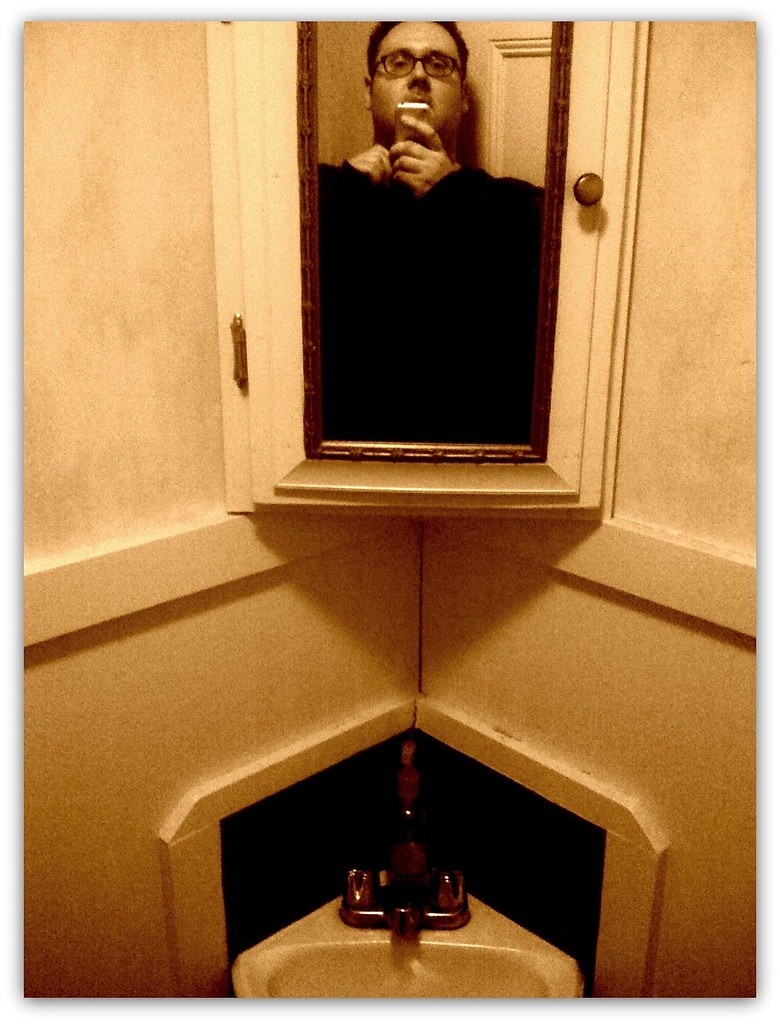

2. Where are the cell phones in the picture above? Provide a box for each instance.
[394,103,430,147]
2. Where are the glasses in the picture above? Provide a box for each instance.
[374,51,464,78]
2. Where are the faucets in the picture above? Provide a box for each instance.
[386,882,429,943]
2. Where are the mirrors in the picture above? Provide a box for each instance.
[298,21,574,464]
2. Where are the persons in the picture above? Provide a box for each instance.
[314,21,547,444]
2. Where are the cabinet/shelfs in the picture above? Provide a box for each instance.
[204,22,652,523]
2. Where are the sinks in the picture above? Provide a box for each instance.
[231,873,583,998]
